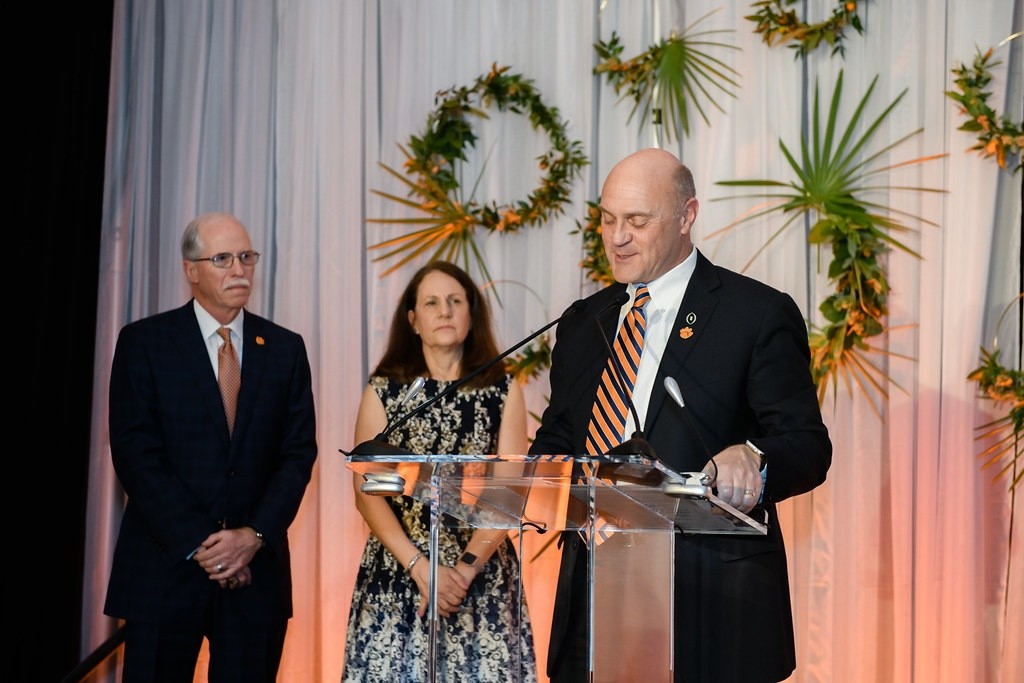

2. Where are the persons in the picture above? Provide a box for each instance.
[529,149,834,683]
[102,210,318,683]
[340,260,537,683]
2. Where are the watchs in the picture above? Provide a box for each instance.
[456,552,481,573]
[745,438,768,472]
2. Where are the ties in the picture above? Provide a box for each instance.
[217,328,241,440]
[578,285,651,548]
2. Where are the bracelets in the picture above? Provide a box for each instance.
[403,551,428,576]
[248,524,266,551]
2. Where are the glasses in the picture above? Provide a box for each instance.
[193,250,261,269]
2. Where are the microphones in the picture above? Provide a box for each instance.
[349,299,588,456]
[594,291,666,487]
[663,375,718,486]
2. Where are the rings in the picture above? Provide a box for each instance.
[216,562,228,571]
[744,490,755,497]
